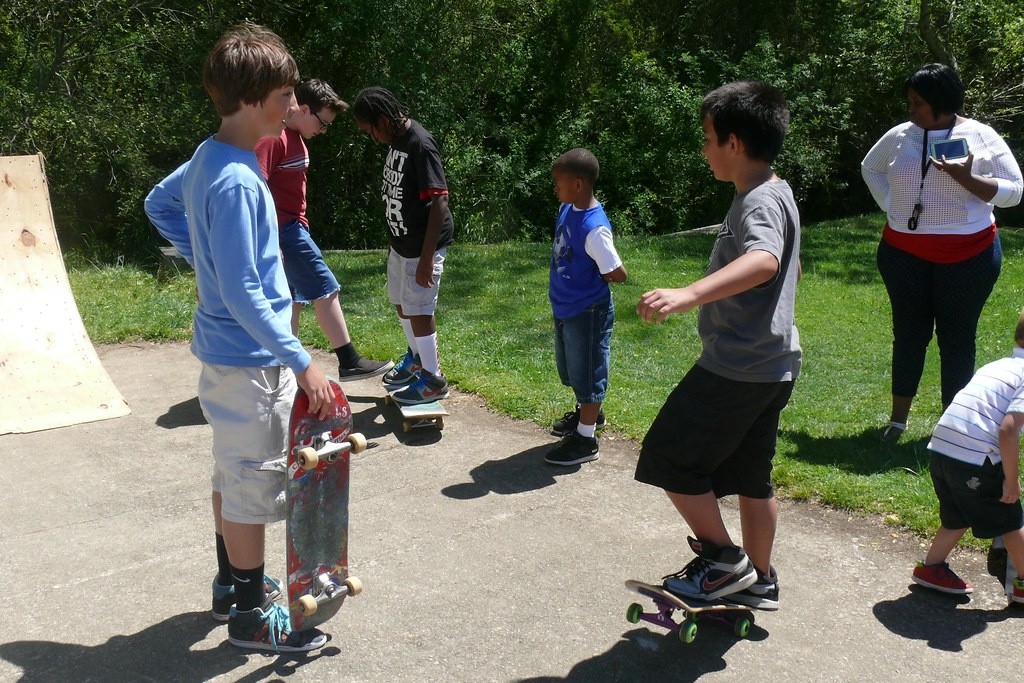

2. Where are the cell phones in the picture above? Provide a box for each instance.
[930,138,969,162]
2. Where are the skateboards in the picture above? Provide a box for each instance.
[283,374,370,632]
[621,578,757,644]
[381,375,450,432]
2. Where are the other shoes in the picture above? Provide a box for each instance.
[882,421,906,440]
[338,358,394,381]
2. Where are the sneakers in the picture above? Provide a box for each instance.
[1012,576,1024,602]
[393,368,449,403]
[212,572,284,620]
[553,404,607,434]
[911,560,973,593]
[661,535,758,600]
[228,594,327,654]
[723,562,780,610]
[383,346,423,384]
[544,429,599,465]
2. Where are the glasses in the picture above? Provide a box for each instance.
[303,103,330,130]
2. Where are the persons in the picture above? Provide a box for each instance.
[144,22,335,652]
[858,63,1023,443]
[252,77,394,380]
[636,80,800,613]
[352,87,454,404]
[543,147,626,466]
[911,309,1024,604]
